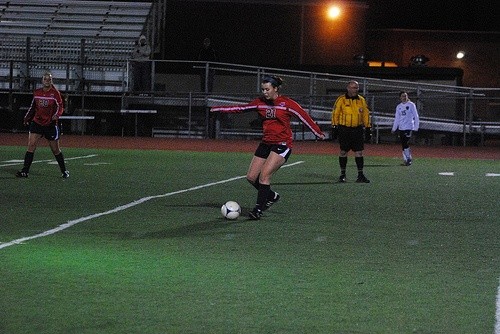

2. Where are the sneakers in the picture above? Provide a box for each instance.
[403,158,411,166]
[61,170,70,178]
[249,204,264,220]
[338,175,346,182]
[356,175,370,182]
[16,171,30,177]
[265,191,280,209]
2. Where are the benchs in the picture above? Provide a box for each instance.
[0,0,154,91]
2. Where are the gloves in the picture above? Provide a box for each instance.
[365,127,373,140]
[331,125,338,140]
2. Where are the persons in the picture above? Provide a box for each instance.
[133,36,151,96]
[390,91,419,166]
[210,76,326,220]
[331,80,372,183]
[198,38,215,92]
[16,72,70,178]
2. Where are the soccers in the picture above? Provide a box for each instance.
[221,201,241,219]
